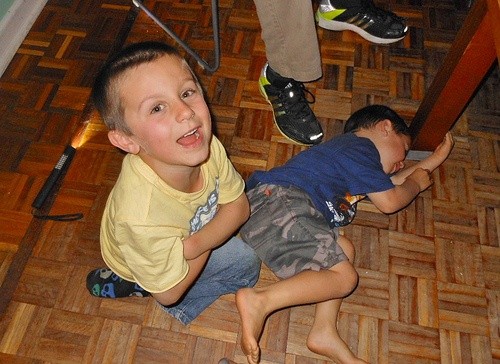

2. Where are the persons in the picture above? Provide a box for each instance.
[253,0,408,146]
[234,105,455,364]
[86,43,260,326]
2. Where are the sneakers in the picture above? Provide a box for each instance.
[259,63,323,146]
[316,1,409,44]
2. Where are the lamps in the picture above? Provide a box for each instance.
[31,145,85,222]
